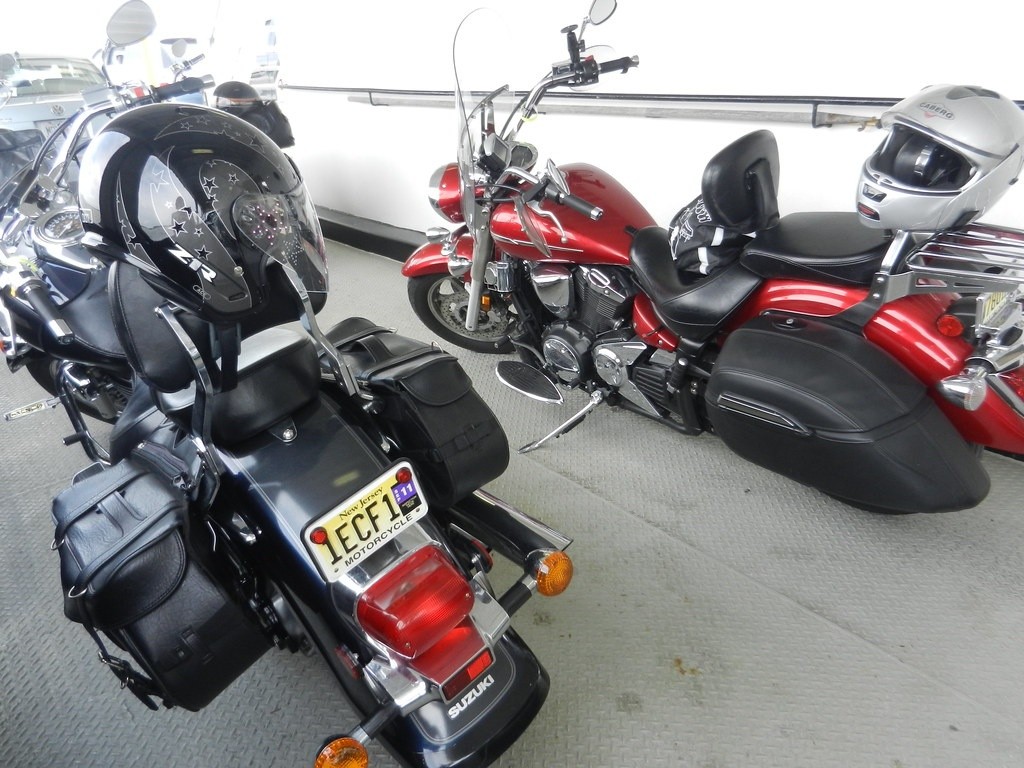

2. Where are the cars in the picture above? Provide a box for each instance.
[0,47,111,144]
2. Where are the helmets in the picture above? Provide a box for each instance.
[75,103,330,326]
[857,84,1024,230]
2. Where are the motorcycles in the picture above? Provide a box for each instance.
[2,0,572,768]
[400,0,1024,517]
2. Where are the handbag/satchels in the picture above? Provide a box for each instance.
[667,195,741,275]
